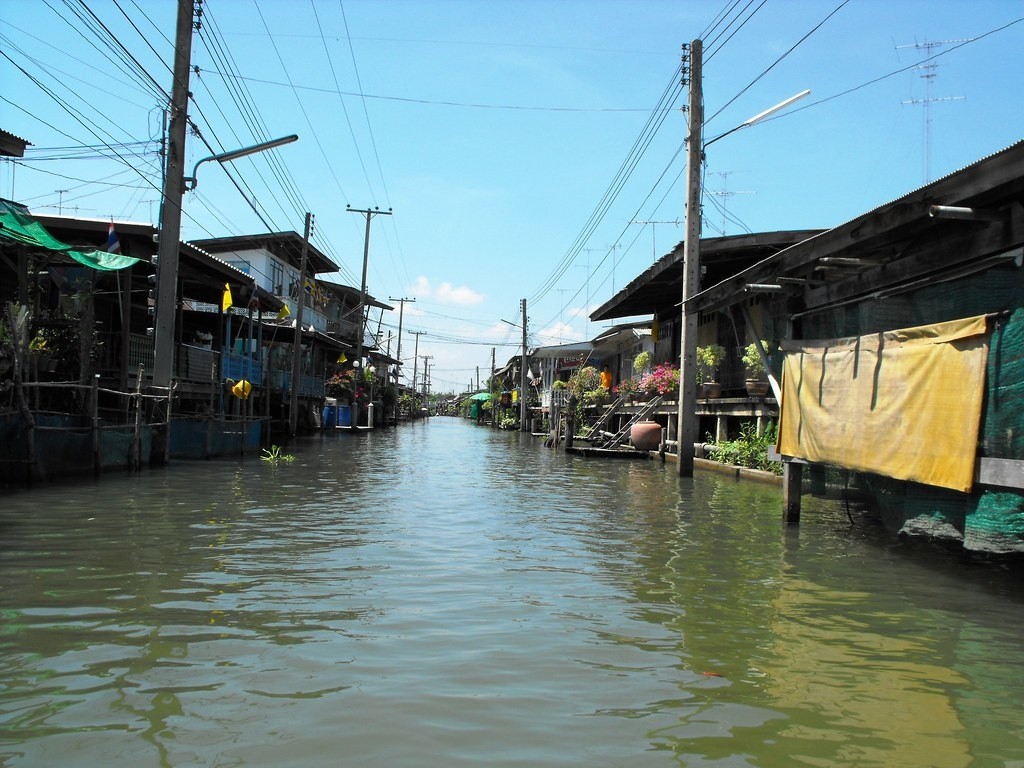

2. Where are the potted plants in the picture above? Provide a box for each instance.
[696,344,727,399]
[25,330,61,372]
[633,351,655,402]
[741,340,770,398]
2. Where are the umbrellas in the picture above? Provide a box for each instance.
[470,392,491,401]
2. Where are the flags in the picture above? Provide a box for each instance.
[107,223,121,254]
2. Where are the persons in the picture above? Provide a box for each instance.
[599,365,612,395]
[364,363,372,382]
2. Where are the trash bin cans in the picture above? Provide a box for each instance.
[323,405,336,428]
[338,405,351,426]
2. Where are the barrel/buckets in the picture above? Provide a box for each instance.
[323,406,336,427]
[339,405,351,425]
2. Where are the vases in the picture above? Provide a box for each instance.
[631,421,662,450]
[644,392,655,401]
[665,392,677,401]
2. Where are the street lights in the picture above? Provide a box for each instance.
[501,318,528,431]
[149,134,300,466]
[676,89,812,479]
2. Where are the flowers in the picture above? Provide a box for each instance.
[324,369,368,406]
[612,378,638,397]
[639,363,681,392]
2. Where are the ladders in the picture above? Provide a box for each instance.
[585,398,625,438]
[602,396,664,450]
